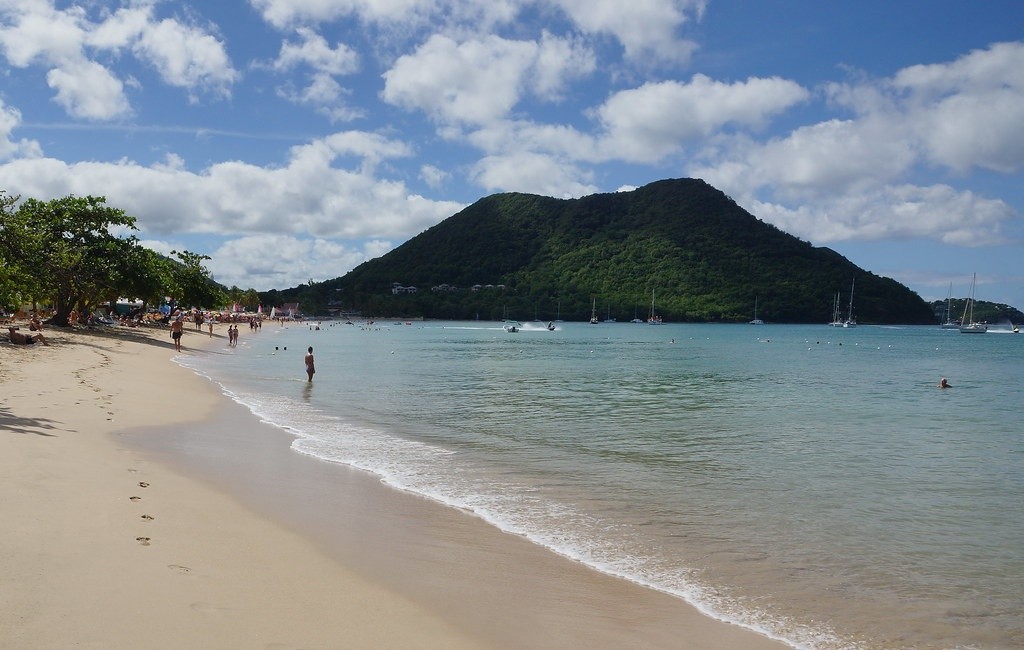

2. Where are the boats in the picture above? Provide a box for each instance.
[503,325,519,333]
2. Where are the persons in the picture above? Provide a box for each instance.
[228,325,238,345]
[70,309,162,327]
[249,318,262,332]
[209,322,213,338]
[937,378,952,388]
[179,310,249,331]
[304,346,315,382]
[9,327,50,346]
[170,317,183,352]
[29,314,45,331]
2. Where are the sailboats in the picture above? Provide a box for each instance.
[647,289,663,325]
[959,272,987,333]
[829,278,857,328]
[749,296,764,325]
[630,299,643,323]
[604,304,616,323]
[590,298,598,324]
[940,282,960,329]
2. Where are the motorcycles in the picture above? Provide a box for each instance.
[1014,328,1020,333]
[545,325,556,331]
